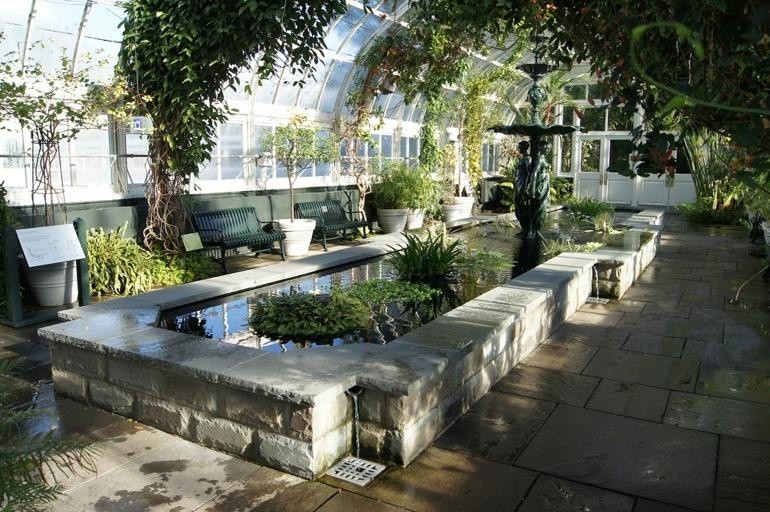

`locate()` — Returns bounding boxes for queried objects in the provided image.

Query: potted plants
[369,154,475,233]
[1,30,161,308]
[259,124,341,256]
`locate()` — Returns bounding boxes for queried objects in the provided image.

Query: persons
[512,139,532,238]
[532,138,555,239]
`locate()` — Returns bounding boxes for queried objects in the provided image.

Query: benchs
[191,207,286,274]
[294,200,369,251]
